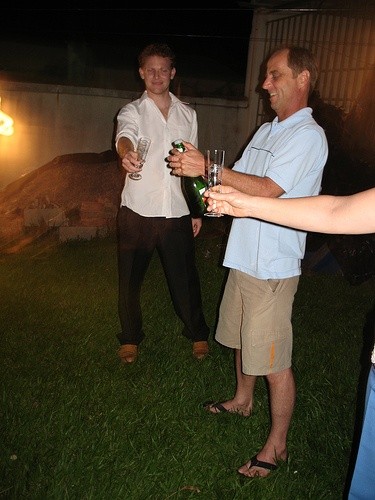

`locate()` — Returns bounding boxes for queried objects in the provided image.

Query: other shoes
[192,341,209,359]
[120,344,137,364]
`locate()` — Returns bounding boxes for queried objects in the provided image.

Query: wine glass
[128,137,152,180]
[204,149,226,216]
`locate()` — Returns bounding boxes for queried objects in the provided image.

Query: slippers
[235,453,290,479]
[202,399,233,415]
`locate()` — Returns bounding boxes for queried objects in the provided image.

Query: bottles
[173,140,209,219]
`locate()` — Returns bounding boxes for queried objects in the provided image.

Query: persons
[165,44,329,482]
[114,43,210,366]
[202,184,375,500]
